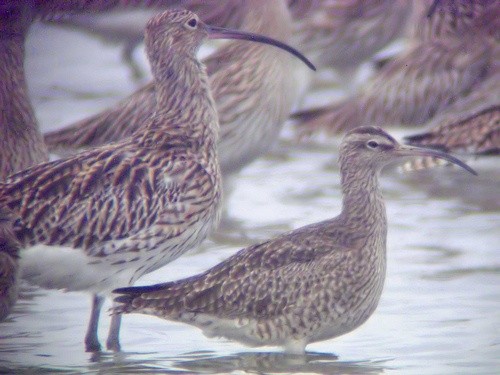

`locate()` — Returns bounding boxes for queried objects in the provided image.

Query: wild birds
[1,2,500,356]
